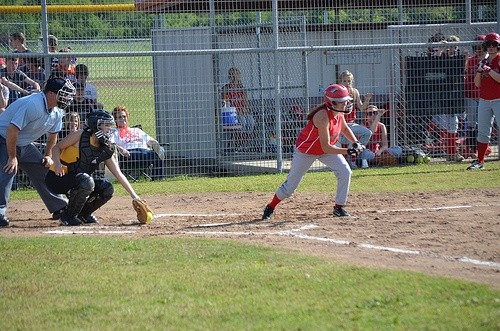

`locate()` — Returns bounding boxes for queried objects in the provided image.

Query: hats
[46,78,69,95]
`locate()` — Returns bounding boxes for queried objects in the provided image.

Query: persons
[358,105,402,159]
[338,69,374,169]
[426,33,492,161]
[44,109,154,226]
[223,68,256,153]
[110,107,166,180]
[0,74,76,226]
[466,33,500,170]
[0,30,97,145]
[262,84,366,220]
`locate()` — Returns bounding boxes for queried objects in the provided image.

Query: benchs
[224,125,243,148]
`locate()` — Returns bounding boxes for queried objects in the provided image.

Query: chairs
[121,124,170,183]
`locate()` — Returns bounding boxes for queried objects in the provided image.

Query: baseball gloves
[131,199,153,224]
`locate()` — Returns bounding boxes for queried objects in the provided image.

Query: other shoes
[0,219,9,225]
[53,205,67,218]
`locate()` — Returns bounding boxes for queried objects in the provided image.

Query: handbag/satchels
[378,147,422,165]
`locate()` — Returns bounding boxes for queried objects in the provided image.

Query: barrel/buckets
[221,107,238,125]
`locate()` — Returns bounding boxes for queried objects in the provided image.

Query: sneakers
[468,162,484,170]
[60,214,82,227]
[333,207,349,217]
[446,154,464,161]
[263,205,274,219]
[77,214,97,224]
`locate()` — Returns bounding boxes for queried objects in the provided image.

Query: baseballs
[407,155,414,162]
[415,157,423,164]
[146,211,152,222]
[424,157,430,162]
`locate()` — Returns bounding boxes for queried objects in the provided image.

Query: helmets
[89,110,114,147]
[472,35,487,51]
[324,85,354,108]
[483,32,500,46]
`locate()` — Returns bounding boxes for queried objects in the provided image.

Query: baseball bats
[352,142,362,150]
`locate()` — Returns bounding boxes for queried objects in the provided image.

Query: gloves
[348,141,365,156]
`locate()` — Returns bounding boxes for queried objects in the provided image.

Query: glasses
[367,111,378,116]
[485,41,499,47]
